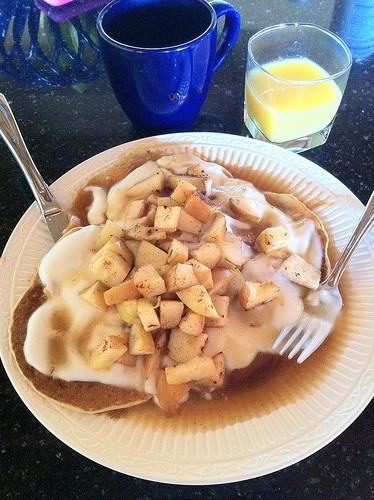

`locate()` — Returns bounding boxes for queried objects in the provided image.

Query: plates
[0,132,374,486]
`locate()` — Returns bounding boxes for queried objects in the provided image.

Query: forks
[271,191,374,365]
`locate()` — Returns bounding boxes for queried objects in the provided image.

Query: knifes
[0,93,70,244]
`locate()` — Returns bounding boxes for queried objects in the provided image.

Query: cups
[96,0,241,136]
[244,22,353,154]
